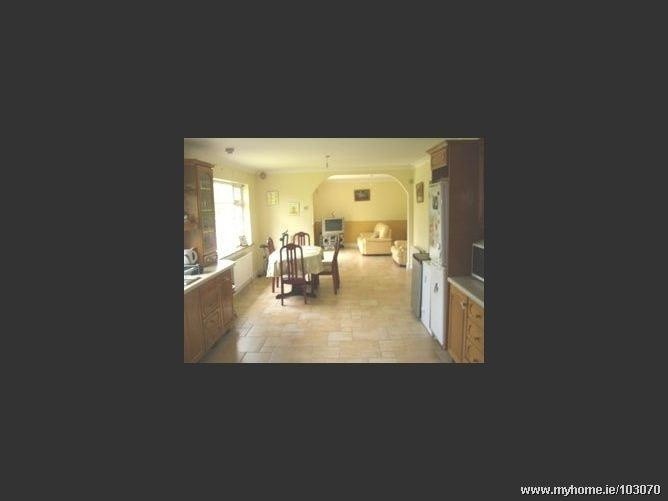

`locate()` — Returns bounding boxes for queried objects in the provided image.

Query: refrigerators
[428,180,449,342]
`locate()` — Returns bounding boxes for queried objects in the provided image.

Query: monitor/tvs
[323,216,344,233]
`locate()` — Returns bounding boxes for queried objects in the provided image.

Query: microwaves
[471,239,484,283]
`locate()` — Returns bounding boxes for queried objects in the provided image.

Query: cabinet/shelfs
[447,284,467,362]
[183,268,232,364]
[184,164,218,269]
[463,299,484,364]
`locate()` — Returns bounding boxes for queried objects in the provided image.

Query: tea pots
[184,248,198,266]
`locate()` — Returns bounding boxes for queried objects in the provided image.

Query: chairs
[266,232,343,308]
[358,223,393,256]
[394,239,407,268]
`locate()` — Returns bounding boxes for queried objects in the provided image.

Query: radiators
[231,252,253,293]
